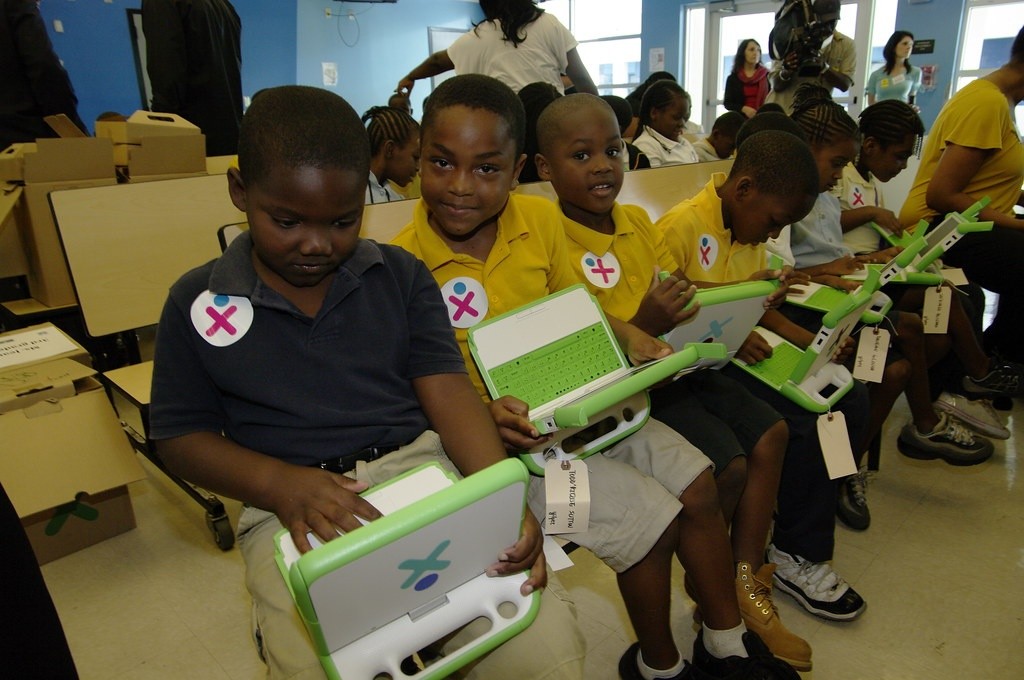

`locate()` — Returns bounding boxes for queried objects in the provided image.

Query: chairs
[1,153,740,666]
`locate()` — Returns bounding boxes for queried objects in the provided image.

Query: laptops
[272,192,994,680]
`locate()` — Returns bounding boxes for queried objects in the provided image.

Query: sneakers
[897,411,996,466]
[838,467,872,532]
[764,541,868,622]
[962,364,1024,397]
[936,392,1013,441]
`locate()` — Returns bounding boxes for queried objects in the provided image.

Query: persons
[142,0,244,157]
[764,0,857,117]
[145,85,585,680]
[357,27,1024,680]
[901,27,1024,359]
[867,31,922,115]
[396,0,598,98]
[723,39,772,118]
[0,0,93,154]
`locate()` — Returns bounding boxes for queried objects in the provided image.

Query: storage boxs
[0,321,148,566]
[0,110,240,309]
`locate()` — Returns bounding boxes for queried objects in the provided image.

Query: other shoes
[619,627,802,680]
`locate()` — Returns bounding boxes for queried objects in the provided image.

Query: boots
[692,554,813,668]
